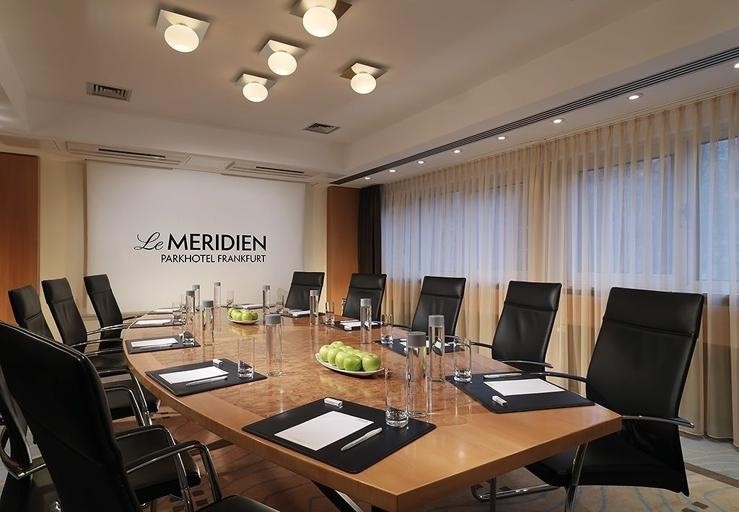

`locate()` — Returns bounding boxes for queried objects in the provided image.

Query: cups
[381,313,394,344]
[325,302,335,327]
[453,336,473,384]
[238,336,254,378]
[386,366,410,429]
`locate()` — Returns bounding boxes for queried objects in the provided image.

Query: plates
[315,352,385,380]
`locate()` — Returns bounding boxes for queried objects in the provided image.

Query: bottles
[265,314,283,375]
[407,333,432,417]
[174,282,283,348]
[429,315,446,382]
[359,297,374,343]
[309,290,319,326]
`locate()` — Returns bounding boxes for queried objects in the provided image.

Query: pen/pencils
[483,372,523,379]
[494,396,509,406]
[340,427,383,452]
[141,345,172,349]
[186,376,228,387]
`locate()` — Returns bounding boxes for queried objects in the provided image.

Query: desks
[116,299,625,510]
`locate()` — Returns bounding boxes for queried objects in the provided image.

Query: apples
[319,340,381,371]
[228,307,258,321]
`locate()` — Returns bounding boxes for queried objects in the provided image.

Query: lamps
[150,5,214,58]
[287,0,354,40]
[233,69,283,105]
[338,57,390,99]
[255,35,309,80]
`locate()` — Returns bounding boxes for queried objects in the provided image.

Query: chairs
[454,278,565,384]
[0,317,291,509]
[79,274,146,350]
[339,272,389,320]
[395,270,469,341]
[0,370,204,510]
[282,268,327,310]
[38,273,136,379]
[6,283,162,426]
[469,284,705,510]
